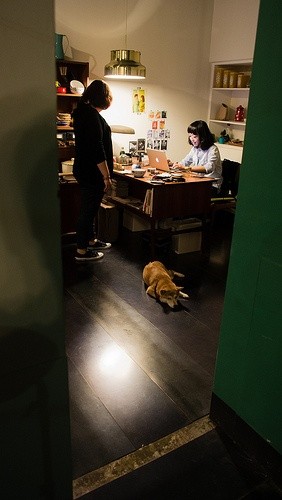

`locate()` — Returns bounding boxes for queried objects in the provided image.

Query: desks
[110,160,217,256]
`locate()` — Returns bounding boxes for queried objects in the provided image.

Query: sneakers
[75,249,104,261]
[88,239,111,249]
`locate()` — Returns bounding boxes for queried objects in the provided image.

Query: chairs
[211,160,241,209]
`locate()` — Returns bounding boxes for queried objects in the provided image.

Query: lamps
[110,125,135,134]
[103,0,146,79]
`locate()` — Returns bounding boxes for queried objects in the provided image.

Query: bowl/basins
[56,112,71,126]
[132,169,146,177]
[69,80,85,95]
[61,157,76,173]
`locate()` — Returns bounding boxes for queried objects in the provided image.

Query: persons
[72,80,114,260]
[171,120,223,194]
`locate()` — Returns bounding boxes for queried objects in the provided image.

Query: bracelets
[103,177,111,180]
[189,166,192,171]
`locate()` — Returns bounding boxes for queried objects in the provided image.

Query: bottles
[120,147,125,156]
[236,104,244,121]
[215,68,250,88]
[214,102,228,120]
[225,123,231,139]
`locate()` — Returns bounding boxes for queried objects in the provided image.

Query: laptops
[146,148,181,173]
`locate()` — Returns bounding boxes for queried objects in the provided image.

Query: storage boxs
[171,218,202,255]
[123,208,151,232]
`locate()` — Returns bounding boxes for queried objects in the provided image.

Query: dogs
[143,261,190,308]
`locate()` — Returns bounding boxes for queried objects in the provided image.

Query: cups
[218,137,224,144]
[56,87,66,94]
[113,155,140,171]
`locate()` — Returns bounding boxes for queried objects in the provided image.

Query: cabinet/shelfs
[207,0,260,164]
[56,60,89,234]
